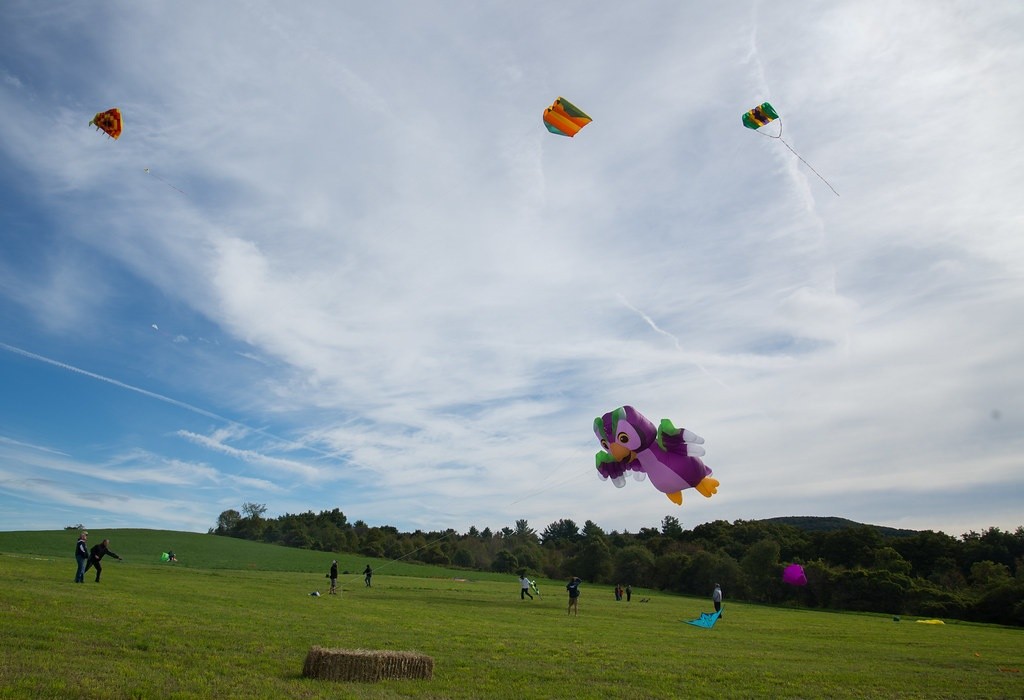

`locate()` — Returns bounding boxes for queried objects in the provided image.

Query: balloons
[593,404,721,506]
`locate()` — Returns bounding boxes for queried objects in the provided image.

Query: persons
[566,576,582,616]
[74,531,88,583]
[84,539,122,582]
[713,583,723,618]
[615,584,632,602]
[363,564,372,587]
[329,560,338,594]
[519,574,534,600]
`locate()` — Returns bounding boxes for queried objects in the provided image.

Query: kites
[742,101,779,130]
[677,603,726,629]
[89,107,122,140]
[529,580,540,596]
[784,564,808,585]
[542,96,593,137]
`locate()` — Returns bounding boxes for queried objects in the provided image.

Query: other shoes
[333,593,336,595]
[329,593,332,594]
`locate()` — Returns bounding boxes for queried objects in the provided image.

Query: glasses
[86,534,88,536]
